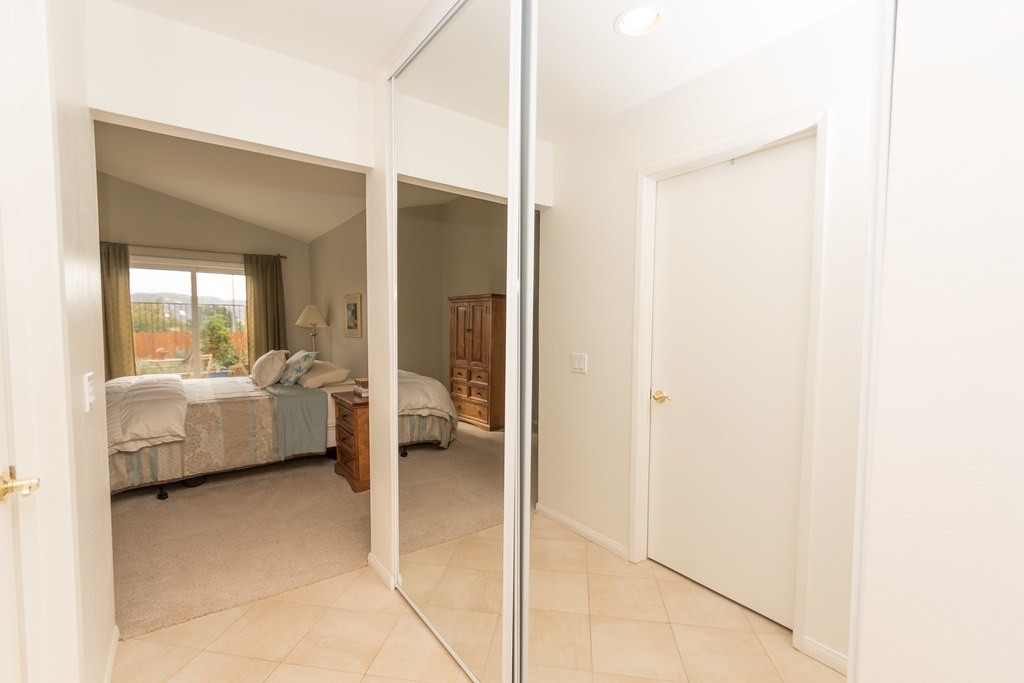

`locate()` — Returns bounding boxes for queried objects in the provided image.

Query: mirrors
[383,0,510,683]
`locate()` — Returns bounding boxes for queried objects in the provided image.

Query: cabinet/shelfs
[446,292,507,435]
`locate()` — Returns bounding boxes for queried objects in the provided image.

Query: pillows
[248,347,290,391]
[280,348,318,388]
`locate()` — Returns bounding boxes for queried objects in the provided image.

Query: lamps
[292,302,331,352]
[614,4,665,43]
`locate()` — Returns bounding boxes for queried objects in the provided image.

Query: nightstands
[330,387,371,496]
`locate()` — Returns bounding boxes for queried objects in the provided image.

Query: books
[353,386,369,398]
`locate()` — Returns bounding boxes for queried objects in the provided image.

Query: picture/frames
[340,291,364,340]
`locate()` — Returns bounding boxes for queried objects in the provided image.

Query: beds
[398,366,463,454]
[105,371,354,500]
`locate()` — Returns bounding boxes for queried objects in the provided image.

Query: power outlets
[82,370,101,409]
[568,354,588,377]
